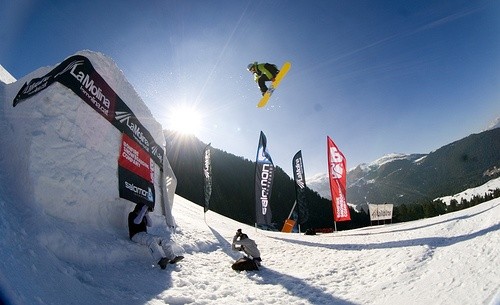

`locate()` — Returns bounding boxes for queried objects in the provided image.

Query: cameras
[236,229,242,236]
[146,203,154,212]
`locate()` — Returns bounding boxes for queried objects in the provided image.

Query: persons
[232,230,262,273]
[128,203,183,270]
[248,62,279,95]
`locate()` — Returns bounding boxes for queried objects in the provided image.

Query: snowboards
[257,62,293,107]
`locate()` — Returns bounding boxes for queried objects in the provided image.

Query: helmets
[247,63,254,71]
[240,234,247,240]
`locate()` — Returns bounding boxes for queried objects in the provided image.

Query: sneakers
[255,260,261,266]
[261,91,265,95]
[255,262,262,270]
[158,258,169,270]
[170,256,184,264]
